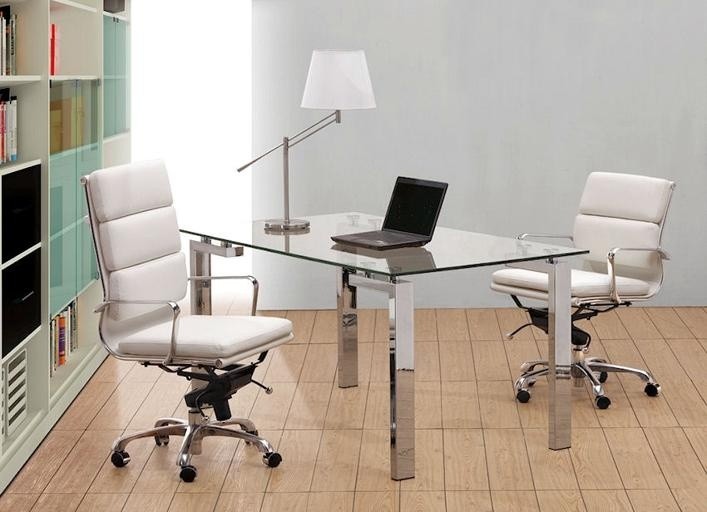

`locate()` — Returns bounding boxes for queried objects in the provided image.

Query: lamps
[237,50,377,233]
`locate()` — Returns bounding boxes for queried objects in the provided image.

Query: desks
[179,212,590,481]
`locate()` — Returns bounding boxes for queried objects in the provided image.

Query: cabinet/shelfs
[0,0,131,495]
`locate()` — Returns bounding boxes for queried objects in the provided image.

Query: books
[50,96,85,154]
[0,13,15,75]
[50,297,79,378]
[1,96,17,164]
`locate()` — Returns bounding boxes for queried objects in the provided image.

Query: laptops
[331,176,448,251]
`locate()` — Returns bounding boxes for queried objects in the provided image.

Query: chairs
[490,171,676,410]
[80,159,295,483]
